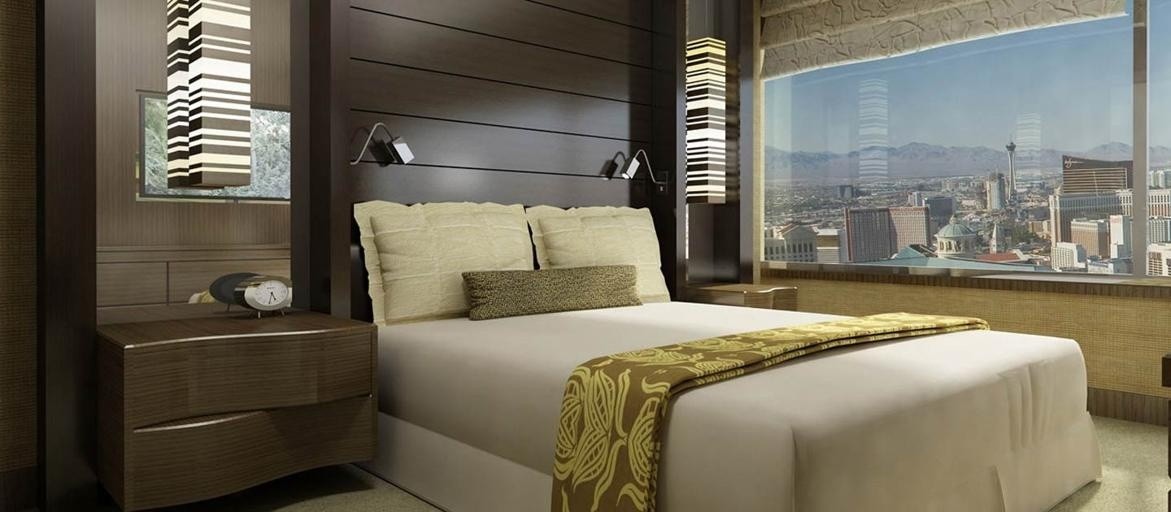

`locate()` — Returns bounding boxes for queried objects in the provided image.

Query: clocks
[235,274,295,320]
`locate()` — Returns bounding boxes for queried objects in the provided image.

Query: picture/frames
[140,96,290,200]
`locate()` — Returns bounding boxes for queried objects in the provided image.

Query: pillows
[525,205,674,306]
[461,265,643,320]
[352,199,533,329]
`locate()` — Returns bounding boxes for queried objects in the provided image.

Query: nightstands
[682,282,799,310]
[95,311,379,512]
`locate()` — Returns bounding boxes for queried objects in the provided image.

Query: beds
[366,304,1104,512]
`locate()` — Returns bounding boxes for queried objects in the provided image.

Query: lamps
[599,151,626,181]
[352,126,393,167]
[620,149,669,195]
[189,0,251,187]
[350,122,414,166]
[165,0,190,188]
[685,37,727,205]
